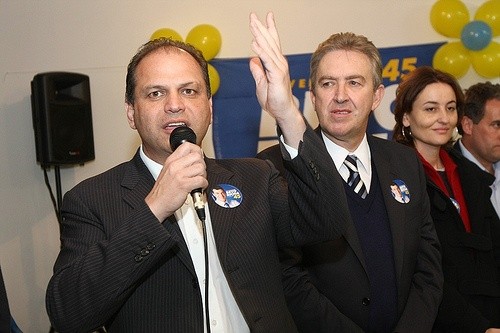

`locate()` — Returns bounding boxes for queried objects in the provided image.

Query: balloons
[150,28,184,44]
[429,0,500,80]
[185,24,222,62]
[207,64,220,95]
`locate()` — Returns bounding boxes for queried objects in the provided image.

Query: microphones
[170,127,205,221]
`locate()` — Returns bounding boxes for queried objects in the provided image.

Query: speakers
[30,71,95,170]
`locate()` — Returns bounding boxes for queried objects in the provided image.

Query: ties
[343,154,368,199]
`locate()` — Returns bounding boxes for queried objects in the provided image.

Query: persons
[210,185,240,207]
[254,32,443,333]
[390,65,500,333]
[46,9,348,333]
[391,182,410,204]
